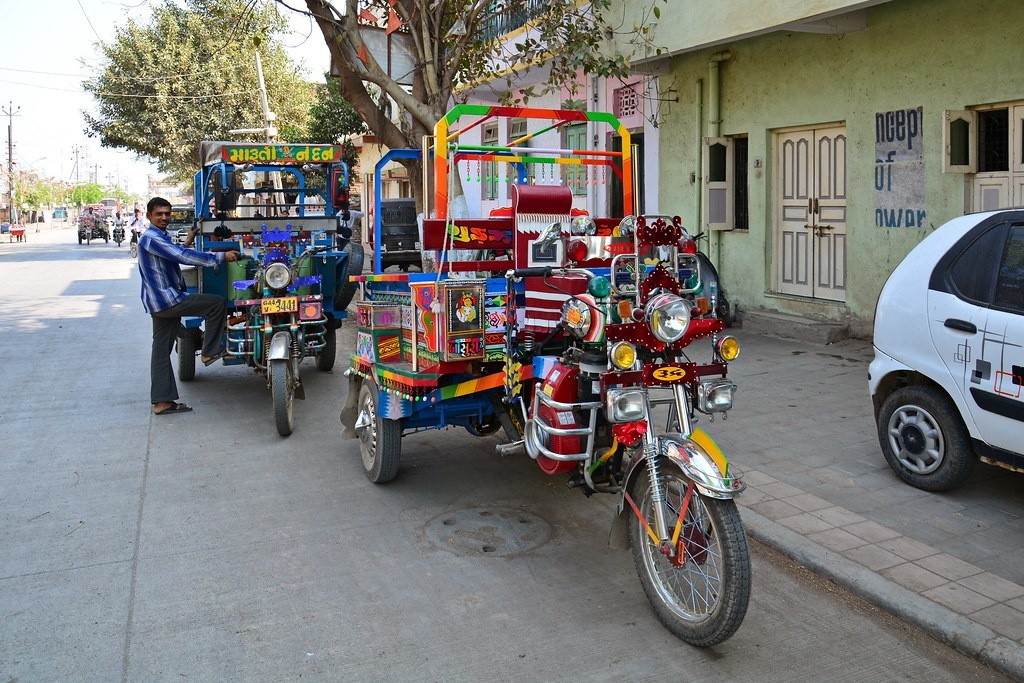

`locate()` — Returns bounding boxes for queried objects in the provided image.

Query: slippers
[156,400,192,414]
[205,349,228,366]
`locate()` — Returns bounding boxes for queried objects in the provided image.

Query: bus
[99,198,118,217]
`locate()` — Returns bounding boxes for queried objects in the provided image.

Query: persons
[112,212,127,241]
[335,205,371,251]
[130,207,148,247]
[85,207,96,220]
[137,197,241,416]
[282,207,300,217]
[16,214,25,242]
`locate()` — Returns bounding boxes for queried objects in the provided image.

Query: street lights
[71,144,84,181]
[0,101,23,197]
[106,172,115,184]
[90,163,102,184]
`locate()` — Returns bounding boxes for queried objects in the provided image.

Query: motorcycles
[110,220,128,248]
[76,209,109,245]
[339,101,751,649]
[175,140,365,439]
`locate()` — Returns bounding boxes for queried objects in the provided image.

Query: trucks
[166,203,194,242]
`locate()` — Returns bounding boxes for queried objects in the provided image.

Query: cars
[868,207,1024,491]
